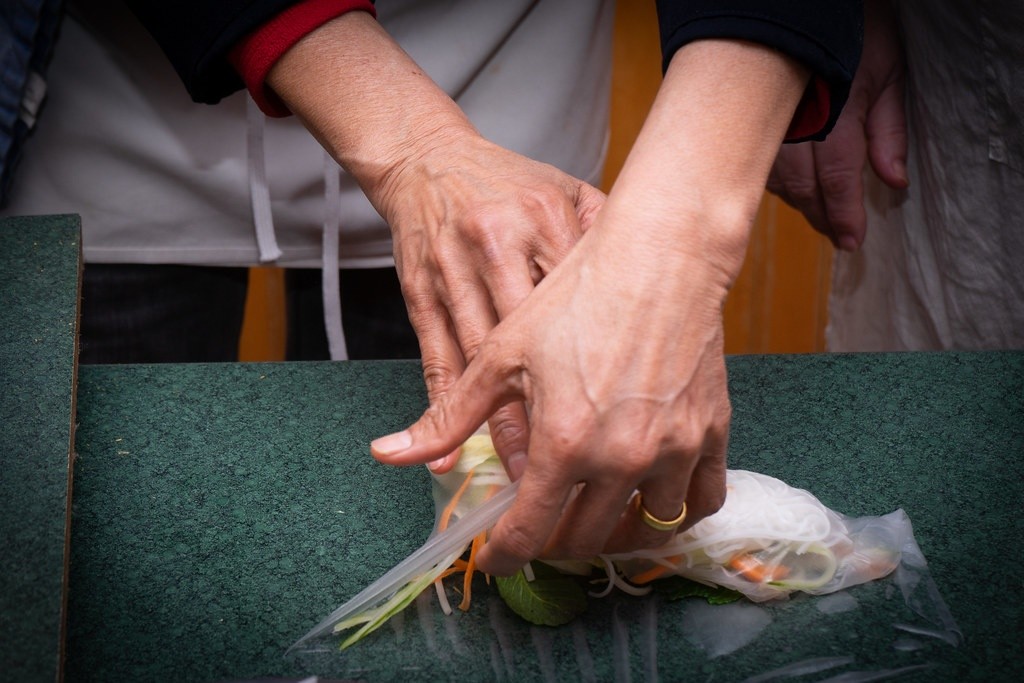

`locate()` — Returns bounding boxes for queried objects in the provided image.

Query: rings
[631,489,687,530]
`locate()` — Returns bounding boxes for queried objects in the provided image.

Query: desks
[0,212,1024,683]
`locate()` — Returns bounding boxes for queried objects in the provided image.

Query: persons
[766,0,1024,354]
[10,2,869,583]
